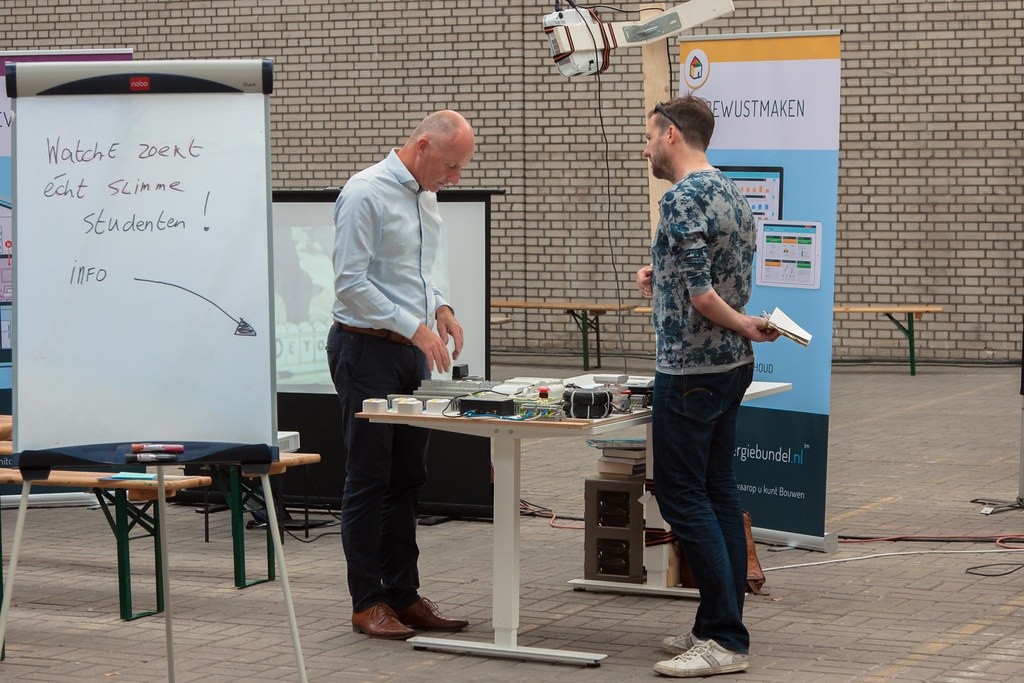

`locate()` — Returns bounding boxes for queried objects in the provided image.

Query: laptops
[243,485,336,530]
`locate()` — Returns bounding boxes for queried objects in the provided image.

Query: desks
[356,374,792,669]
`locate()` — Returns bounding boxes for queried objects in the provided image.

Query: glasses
[653,101,683,132]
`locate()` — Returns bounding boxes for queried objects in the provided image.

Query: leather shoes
[351,600,416,639]
[398,595,468,631]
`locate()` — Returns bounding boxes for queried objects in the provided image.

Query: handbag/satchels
[678,509,770,596]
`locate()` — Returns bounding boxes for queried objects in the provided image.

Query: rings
[644,288,647,295]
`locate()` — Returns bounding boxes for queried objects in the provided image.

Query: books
[761,306,813,347]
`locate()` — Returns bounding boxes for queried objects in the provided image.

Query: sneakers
[653,638,750,677]
[661,629,700,653]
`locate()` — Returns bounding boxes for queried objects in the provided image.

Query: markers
[128,442,184,452]
[125,453,179,462]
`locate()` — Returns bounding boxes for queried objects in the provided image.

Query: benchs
[490,300,641,371]
[0,414,321,621]
[635,306,944,376]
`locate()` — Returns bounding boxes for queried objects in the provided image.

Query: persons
[325,110,475,639]
[635,95,780,677]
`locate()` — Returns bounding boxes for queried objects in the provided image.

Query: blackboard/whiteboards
[10,89,282,470]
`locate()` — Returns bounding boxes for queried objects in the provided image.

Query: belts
[334,320,415,347]
